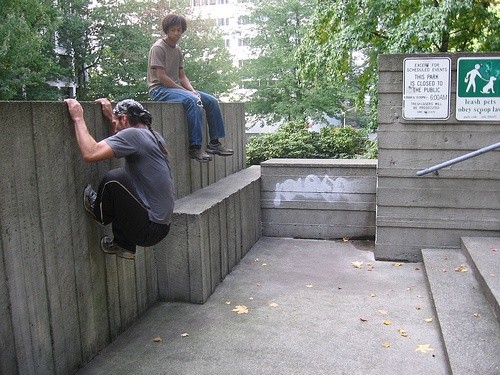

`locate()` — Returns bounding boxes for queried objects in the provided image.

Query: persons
[66,97,173,259]
[146,14,234,162]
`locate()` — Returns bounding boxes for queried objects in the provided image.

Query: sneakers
[206,142,234,156]
[189,146,212,161]
[83,185,104,226]
[101,235,137,260]
